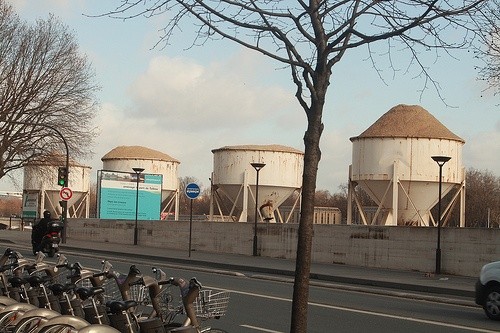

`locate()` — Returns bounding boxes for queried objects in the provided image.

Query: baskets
[0,258,72,289]
[125,273,151,303]
[151,286,182,326]
[184,289,229,318]
[81,271,123,305]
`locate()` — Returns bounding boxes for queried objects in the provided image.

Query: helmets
[43,211,51,218]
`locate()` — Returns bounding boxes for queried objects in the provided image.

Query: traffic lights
[59,201,65,208]
[57,166,67,186]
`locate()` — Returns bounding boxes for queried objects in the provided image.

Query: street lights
[431,155,452,275]
[132,167,145,246]
[249,161,266,259]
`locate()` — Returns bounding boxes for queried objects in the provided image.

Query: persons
[32,211,54,252]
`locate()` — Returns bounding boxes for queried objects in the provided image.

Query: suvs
[473,262,500,321]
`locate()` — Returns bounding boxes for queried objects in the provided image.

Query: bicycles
[0,247,230,333]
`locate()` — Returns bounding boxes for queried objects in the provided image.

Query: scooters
[31,220,63,259]
